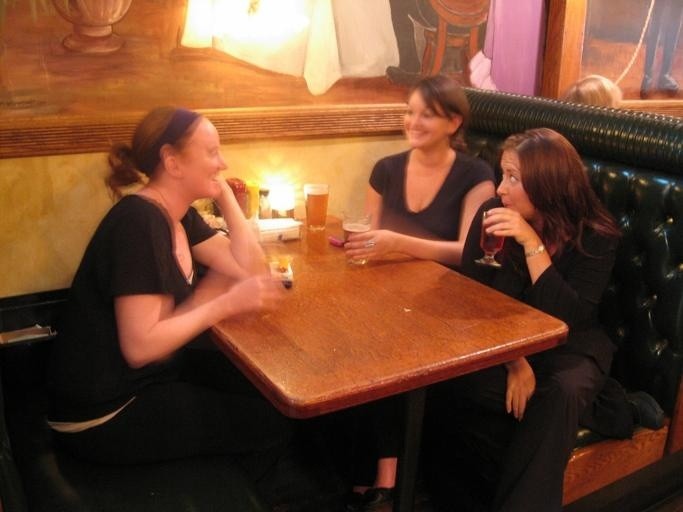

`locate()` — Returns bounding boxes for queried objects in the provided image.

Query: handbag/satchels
[580,378,664,437]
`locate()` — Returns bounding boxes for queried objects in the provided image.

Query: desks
[189,215,570,512]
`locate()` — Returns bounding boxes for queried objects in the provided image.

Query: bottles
[229,178,272,219]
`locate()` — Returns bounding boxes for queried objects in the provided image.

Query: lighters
[275,255,291,272]
[328,235,344,248]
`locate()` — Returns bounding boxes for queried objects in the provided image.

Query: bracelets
[523,243,545,258]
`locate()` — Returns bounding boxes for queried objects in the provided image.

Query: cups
[303,183,330,231]
[340,221,371,267]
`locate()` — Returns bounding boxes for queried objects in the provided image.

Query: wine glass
[475,211,507,268]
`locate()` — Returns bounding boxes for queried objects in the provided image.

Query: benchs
[455,85,683,512]
[0,324,271,512]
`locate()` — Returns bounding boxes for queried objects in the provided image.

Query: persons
[561,73,625,111]
[345,69,496,511]
[42,103,346,510]
[379,0,486,90]
[637,0,682,101]
[418,128,623,510]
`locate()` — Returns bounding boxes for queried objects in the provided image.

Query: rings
[363,238,375,248]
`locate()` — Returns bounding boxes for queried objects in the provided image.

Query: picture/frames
[540,0,683,117]
